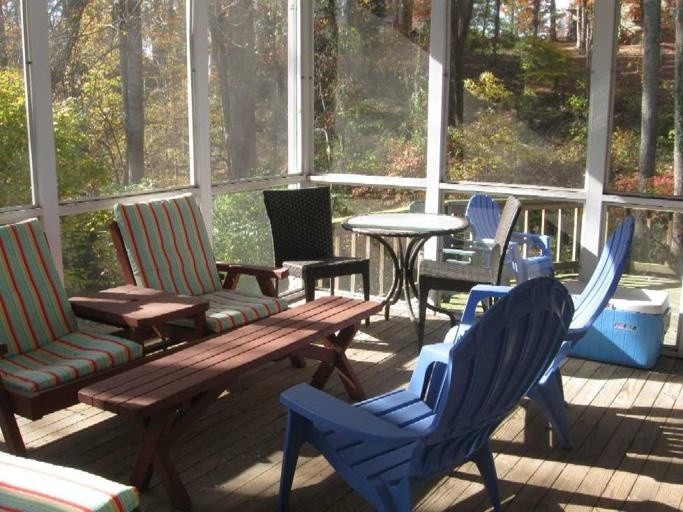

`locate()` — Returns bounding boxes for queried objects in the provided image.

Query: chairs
[104,192,307,413]
[406,214,637,450]
[416,196,523,355]
[262,186,372,329]
[276,275,574,512]
[465,193,556,289]
[2,450,141,511]
[0,217,146,458]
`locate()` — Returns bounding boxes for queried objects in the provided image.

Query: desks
[340,213,470,327]
[67,285,211,427]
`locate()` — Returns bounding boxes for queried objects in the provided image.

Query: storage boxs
[561,279,672,370]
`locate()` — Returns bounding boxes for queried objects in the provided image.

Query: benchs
[78,293,383,510]
